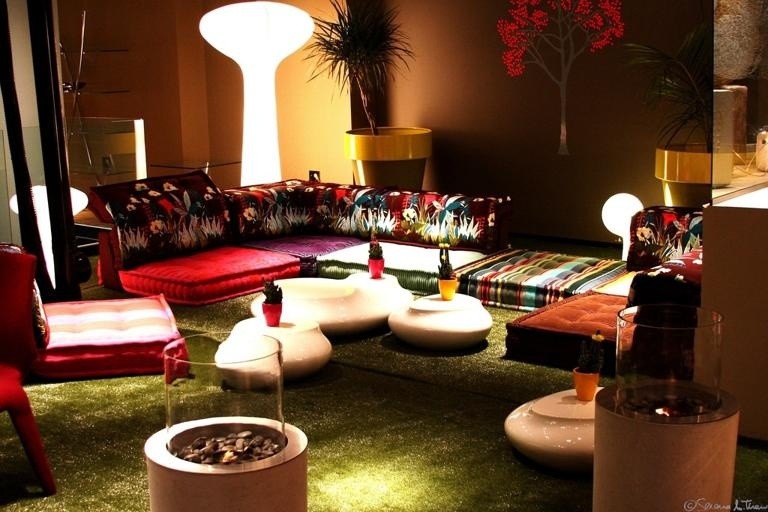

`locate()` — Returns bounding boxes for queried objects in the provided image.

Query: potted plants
[303,0,433,191]
[620,12,713,210]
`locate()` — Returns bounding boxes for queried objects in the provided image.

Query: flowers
[576,328,604,371]
[436,242,457,280]
[259,276,285,303]
[368,236,385,259]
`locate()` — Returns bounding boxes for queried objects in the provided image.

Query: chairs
[0,245,60,497]
[5,245,189,407]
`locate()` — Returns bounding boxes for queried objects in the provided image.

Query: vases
[262,303,283,329]
[438,281,460,302]
[367,257,385,280]
[575,368,598,402]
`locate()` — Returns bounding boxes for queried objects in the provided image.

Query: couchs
[452,204,705,375]
[86,169,304,311]
[318,189,511,295]
[228,177,381,275]
